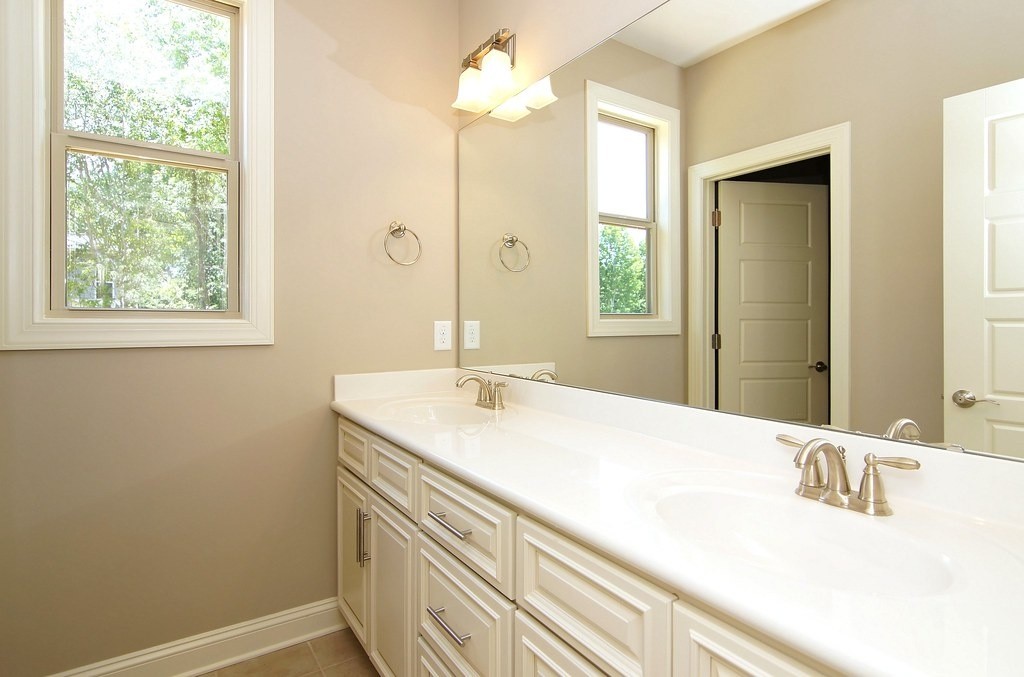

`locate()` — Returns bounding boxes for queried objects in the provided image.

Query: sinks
[370,394,513,436]
[644,484,884,580]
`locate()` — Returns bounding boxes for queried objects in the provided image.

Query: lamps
[488,72,559,124]
[451,27,518,114]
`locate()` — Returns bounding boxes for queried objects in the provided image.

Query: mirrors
[455,0,1024,462]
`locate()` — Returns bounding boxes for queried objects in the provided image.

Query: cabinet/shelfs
[336,415,422,677]
[515,515,679,677]
[672,599,827,676]
[417,461,517,677]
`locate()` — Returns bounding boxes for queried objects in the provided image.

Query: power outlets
[433,320,451,351]
[464,321,480,350]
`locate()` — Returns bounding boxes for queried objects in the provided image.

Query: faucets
[792,438,853,501]
[883,415,920,441]
[453,372,492,406]
[529,367,557,383]
